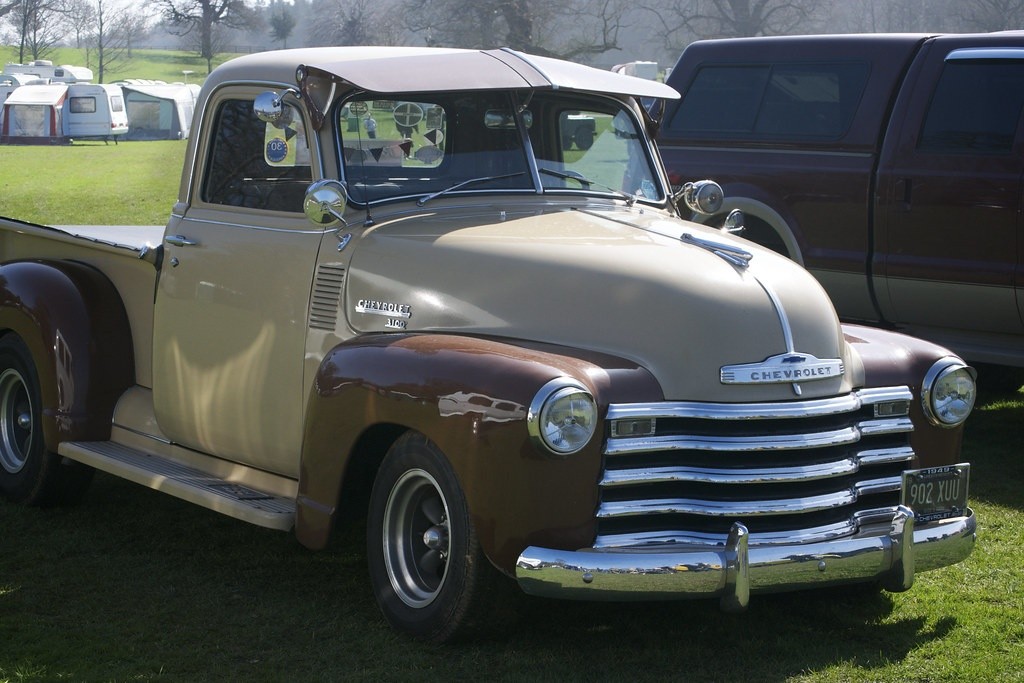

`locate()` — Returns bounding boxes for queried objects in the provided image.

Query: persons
[610,64,660,202]
[364,113,377,139]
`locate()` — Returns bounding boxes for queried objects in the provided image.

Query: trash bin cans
[346,117,359,133]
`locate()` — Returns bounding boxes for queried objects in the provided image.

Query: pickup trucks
[0,44,981,644]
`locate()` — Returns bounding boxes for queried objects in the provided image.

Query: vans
[622,31,1024,373]
